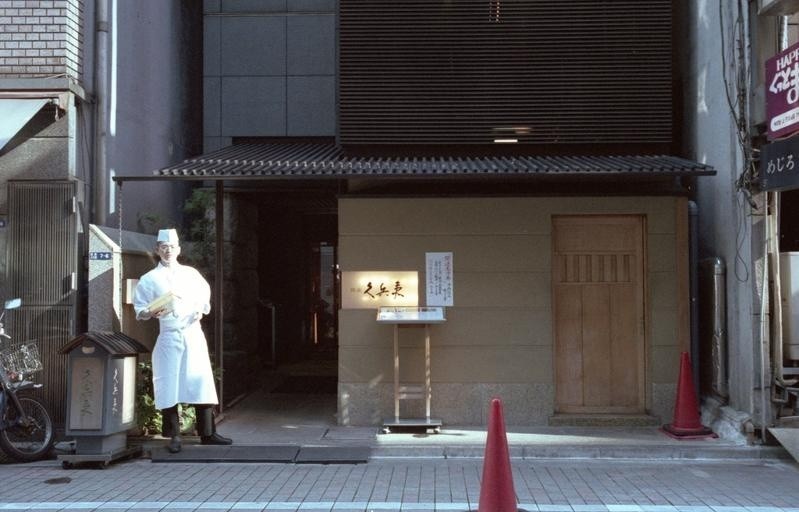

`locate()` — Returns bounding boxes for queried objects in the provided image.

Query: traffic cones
[664,350,713,435]
[478,398,519,512]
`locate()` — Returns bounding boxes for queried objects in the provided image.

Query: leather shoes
[169,439,181,452]
[201,432,232,445]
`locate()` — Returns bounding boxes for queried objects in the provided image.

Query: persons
[132,227,233,453]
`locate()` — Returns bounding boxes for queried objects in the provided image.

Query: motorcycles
[0,297,55,461]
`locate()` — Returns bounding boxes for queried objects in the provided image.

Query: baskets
[0,339,43,381]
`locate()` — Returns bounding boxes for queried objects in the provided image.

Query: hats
[157,229,179,242]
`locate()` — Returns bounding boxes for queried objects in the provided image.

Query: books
[144,291,183,314]
